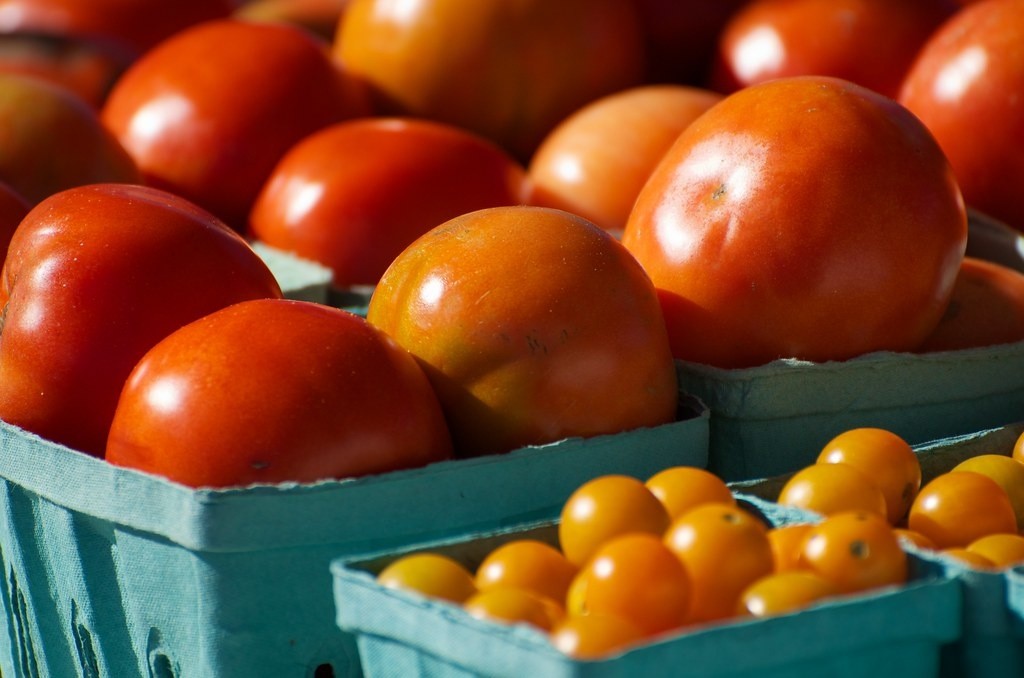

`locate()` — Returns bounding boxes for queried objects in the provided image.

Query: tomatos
[773,427,1024,576]
[368,463,906,661]
[0,0,1024,491]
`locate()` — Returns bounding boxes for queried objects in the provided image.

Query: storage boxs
[0,210,1024,678]
